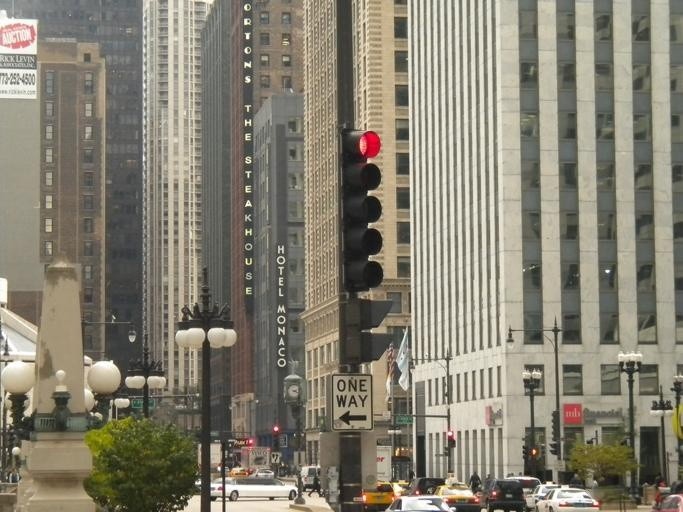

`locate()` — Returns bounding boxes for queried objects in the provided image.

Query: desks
[172,263,239,511]
[648,383,674,487]
[0,312,168,511]
[501,313,565,482]
[519,367,540,477]
[616,349,643,499]
[404,348,459,477]
[383,423,403,487]
[670,372,682,452]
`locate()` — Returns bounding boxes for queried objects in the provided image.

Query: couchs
[521,442,529,459]
[443,447,448,456]
[531,445,537,457]
[271,424,280,433]
[547,441,560,461]
[334,128,386,299]
[549,410,561,442]
[447,430,454,441]
[245,437,255,445]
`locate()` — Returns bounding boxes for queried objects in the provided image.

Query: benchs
[396,329,409,390]
[386,344,395,401]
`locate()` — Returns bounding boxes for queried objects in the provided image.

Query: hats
[362,475,602,512]
[652,489,682,512]
[193,463,321,501]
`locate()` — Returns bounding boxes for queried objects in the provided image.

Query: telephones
[330,375,374,434]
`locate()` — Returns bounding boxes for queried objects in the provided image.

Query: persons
[468,471,481,490]
[308,476,322,497]
[407,467,415,486]
[482,473,491,488]
